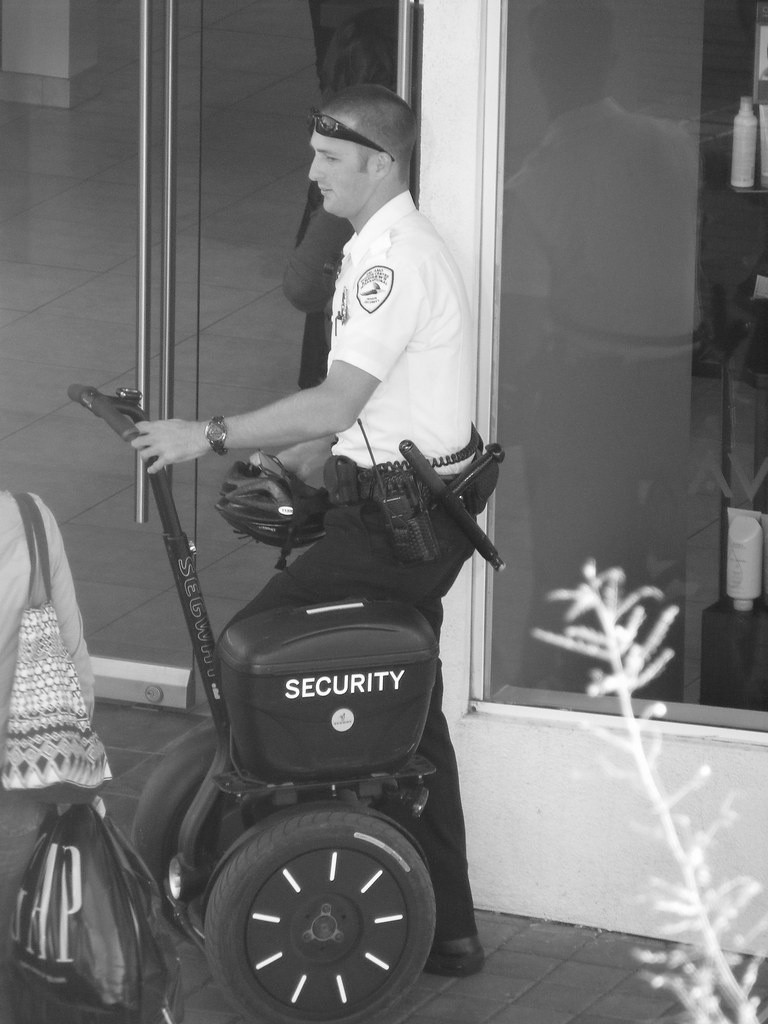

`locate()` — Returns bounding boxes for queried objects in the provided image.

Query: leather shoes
[421,933,485,976]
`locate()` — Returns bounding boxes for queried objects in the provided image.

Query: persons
[288,1,719,699]
[0,491,121,1007]
[132,84,485,980]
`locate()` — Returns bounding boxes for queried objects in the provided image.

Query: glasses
[304,107,396,162]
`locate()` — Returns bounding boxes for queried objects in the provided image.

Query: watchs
[204,416,228,454]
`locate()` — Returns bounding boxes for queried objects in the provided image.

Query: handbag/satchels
[12,802,167,1024]
[1,492,113,807]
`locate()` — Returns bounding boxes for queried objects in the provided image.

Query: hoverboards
[67,380,443,1024]
